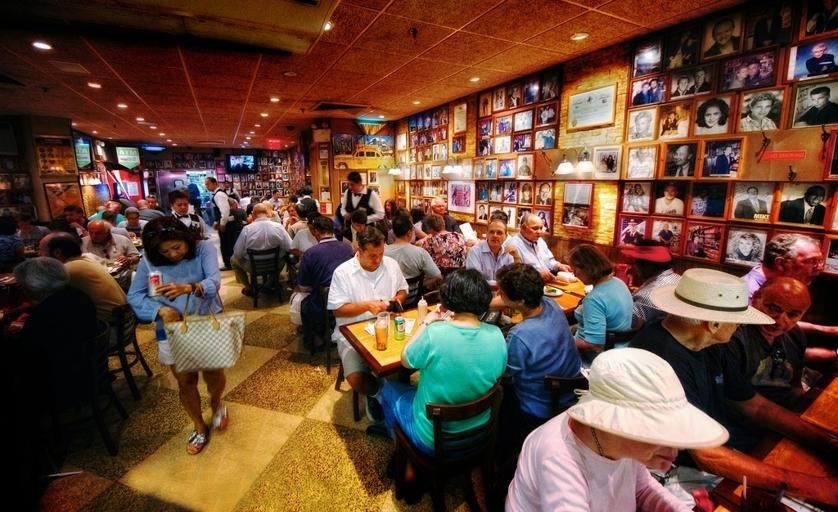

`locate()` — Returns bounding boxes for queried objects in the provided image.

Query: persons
[704,16,739,57]
[685,68,711,93]
[745,63,771,89]
[1,194,164,465]
[205,177,241,271]
[694,99,729,135]
[395,69,589,234]
[632,111,652,140]
[724,63,749,89]
[128,217,229,455]
[794,86,837,125]
[671,144,693,177]
[741,92,778,130]
[661,105,690,138]
[232,170,681,482]
[632,83,652,103]
[669,33,697,68]
[804,43,838,75]
[508,233,836,512]
[645,79,663,101]
[167,190,218,240]
[714,145,734,176]
[217,152,301,200]
[671,74,689,96]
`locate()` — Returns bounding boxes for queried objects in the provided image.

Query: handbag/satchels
[164,311,245,372]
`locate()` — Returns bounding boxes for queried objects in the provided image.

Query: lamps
[388,162,403,176]
[442,155,464,174]
[555,152,592,175]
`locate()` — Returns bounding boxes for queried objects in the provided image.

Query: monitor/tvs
[225,153,258,173]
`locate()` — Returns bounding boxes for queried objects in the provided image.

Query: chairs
[89,302,152,456]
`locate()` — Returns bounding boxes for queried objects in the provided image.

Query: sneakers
[385,450,408,478]
[242,288,261,298]
[220,267,232,271]
[365,425,388,436]
[365,396,384,422]
[303,337,321,349]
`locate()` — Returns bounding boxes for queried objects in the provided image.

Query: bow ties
[354,193,361,196]
[177,216,188,218]
[211,193,216,196]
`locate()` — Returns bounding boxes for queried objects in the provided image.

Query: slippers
[186,423,209,455]
[211,403,228,431]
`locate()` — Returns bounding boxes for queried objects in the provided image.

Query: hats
[650,268,777,326]
[618,246,672,262]
[567,348,730,450]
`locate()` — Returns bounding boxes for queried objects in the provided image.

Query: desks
[712,374,838,512]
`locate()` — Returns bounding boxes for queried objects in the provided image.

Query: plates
[542,285,562,297]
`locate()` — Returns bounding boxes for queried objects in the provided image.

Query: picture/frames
[591,146,623,180]
[566,83,618,133]
[561,183,595,229]
[472,67,562,236]
[452,102,468,153]
[408,103,448,215]
[320,150,328,159]
[397,180,406,209]
[447,180,475,215]
[340,170,381,204]
[144,150,311,203]
[396,132,407,162]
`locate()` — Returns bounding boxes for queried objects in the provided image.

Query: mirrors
[317,142,333,216]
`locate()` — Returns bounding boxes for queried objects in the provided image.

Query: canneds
[395,316,405,342]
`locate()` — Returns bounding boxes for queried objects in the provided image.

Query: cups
[373,312,391,351]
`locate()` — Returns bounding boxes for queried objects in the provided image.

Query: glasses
[142,228,175,239]
[103,247,111,259]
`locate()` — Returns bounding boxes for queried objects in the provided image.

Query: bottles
[416,296,427,318]
[394,303,405,342]
